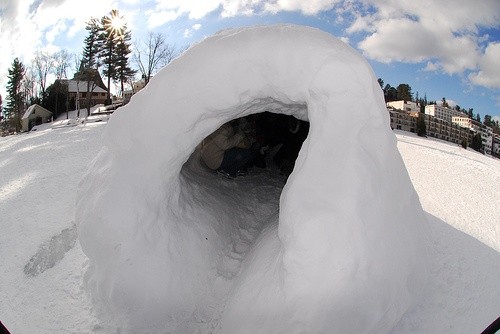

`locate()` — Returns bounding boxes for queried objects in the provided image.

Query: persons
[201,111,309,181]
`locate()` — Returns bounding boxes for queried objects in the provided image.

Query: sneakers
[217,170,233,180]
[236,170,248,176]
